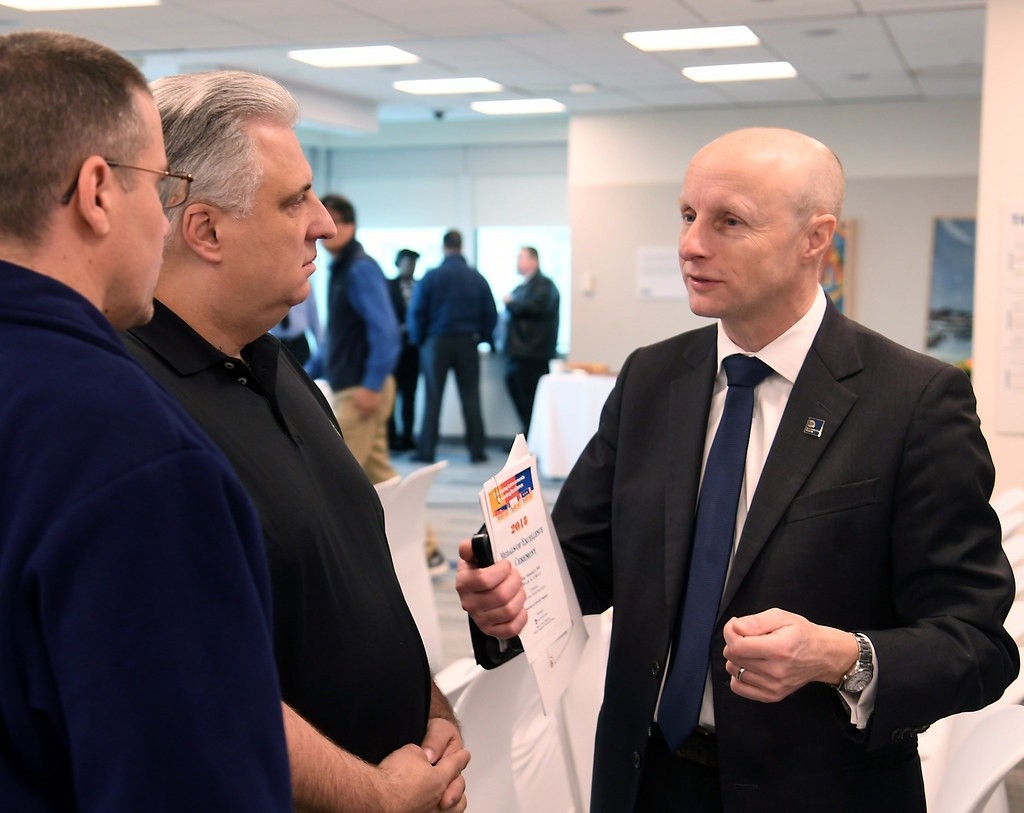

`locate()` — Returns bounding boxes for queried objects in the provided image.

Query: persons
[0,29,293,813]
[320,194,450,577]
[110,70,470,813]
[456,127,1020,813]
[267,283,322,367]
[503,248,559,441]
[385,249,419,451]
[406,232,497,463]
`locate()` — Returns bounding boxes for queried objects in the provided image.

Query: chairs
[917,647,1024,760]
[453,652,585,813]
[929,706,1024,813]
[1002,488,1024,643]
[373,458,455,672]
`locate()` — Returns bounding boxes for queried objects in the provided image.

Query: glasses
[61,160,197,208]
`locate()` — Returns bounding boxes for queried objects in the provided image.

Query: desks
[527,370,617,481]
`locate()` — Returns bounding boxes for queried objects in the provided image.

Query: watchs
[837,633,875,695]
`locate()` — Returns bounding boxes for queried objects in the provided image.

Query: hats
[395,249,420,266]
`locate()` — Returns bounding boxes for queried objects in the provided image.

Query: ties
[657,354,775,742]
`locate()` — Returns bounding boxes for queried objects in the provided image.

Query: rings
[736,668,745,680]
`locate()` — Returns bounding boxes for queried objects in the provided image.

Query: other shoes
[401,438,414,449]
[409,455,432,463]
[472,454,486,463]
